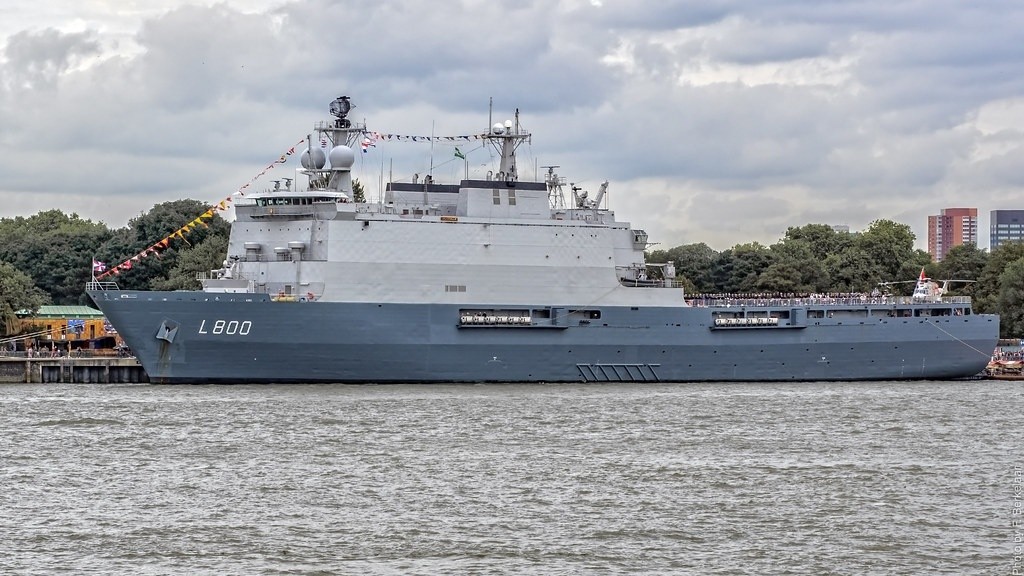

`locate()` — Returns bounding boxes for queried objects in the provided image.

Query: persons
[0,344,82,358]
[684,292,881,307]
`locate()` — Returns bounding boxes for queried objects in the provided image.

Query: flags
[362,143,368,154]
[454,147,464,160]
[365,137,376,148]
[94,258,107,272]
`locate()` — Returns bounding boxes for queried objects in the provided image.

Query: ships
[85,95,1001,388]
